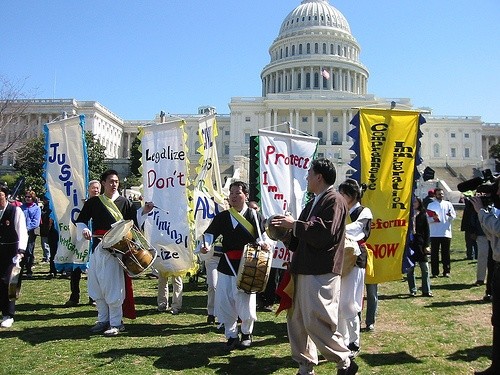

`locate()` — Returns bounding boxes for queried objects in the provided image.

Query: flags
[321,67,330,80]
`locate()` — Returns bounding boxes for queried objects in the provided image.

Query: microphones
[457,177,483,192]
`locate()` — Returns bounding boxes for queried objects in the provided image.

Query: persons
[272,159,359,375]
[75,170,154,337]
[192,181,274,351]
[0,158,456,375]
[461,175,500,375]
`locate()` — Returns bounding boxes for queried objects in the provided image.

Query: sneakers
[91,321,125,335]
[170,306,180,315]
[157,304,169,311]
[0,315,15,328]
[206,315,217,324]
[336,356,358,375]
[224,333,252,350]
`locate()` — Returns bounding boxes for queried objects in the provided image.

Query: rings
[279,221,282,225]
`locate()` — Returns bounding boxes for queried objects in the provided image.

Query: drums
[234,242,275,295]
[8,266,22,301]
[265,214,289,242]
[100,219,159,277]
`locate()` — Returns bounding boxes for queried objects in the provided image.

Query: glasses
[25,194,32,199]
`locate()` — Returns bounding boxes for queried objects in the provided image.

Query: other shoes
[422,293,434,297]
[348,342,362,358]
[483,293,493,302]
[366,324,374,331]
[63,299,79,307]
[429,275,440,278]
[474,280,485,287]
[443,273,452,278]
[464,257,478,260]
[409,292,415,295]
